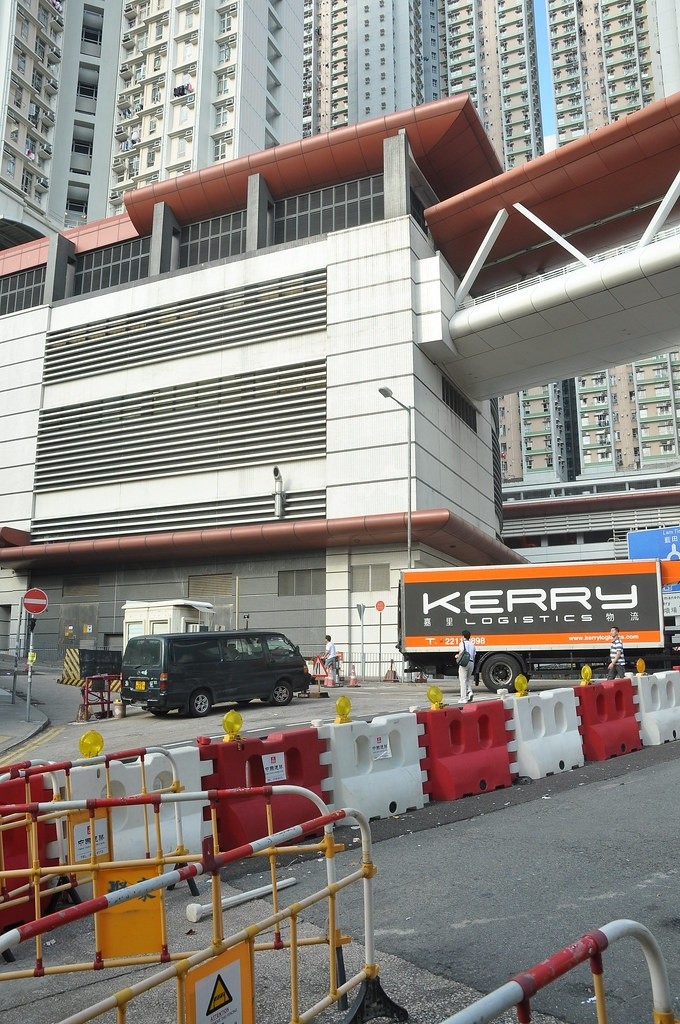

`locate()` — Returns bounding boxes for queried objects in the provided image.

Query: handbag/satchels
[456,642,470,667]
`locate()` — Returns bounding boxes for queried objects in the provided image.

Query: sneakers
[458,699,467,704]
[468,693,475,701]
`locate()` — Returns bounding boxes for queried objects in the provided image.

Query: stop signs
[23,586,49,615]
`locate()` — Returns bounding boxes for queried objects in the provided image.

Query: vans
[119,628,312,719]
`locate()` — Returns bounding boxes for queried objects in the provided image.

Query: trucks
[394,557,680,694]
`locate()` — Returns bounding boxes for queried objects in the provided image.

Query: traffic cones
[326,663,336,688]
[320,665,328,687]
[348,665,361,687]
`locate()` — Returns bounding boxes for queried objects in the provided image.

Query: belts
[469,660,473,662]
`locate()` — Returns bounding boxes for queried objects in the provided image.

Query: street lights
[377,386,412,569]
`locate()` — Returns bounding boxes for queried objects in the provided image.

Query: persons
[607,627,625,680]
[455,630,476,705]
[323,635,336,668]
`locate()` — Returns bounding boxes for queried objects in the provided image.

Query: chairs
[226,644,242,661]
